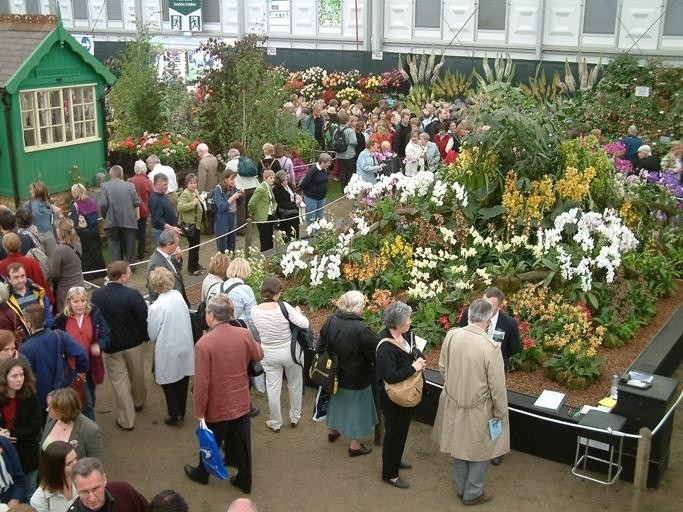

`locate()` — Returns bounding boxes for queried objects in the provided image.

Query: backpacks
[205,184,223,216]
[333,127,347,152]
[260,159,275,180]
[236,157,259,177]
[290,318,314,362]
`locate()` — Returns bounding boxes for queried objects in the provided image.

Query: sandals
[190,265,206,276]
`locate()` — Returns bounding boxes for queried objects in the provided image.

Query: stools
[570,409,630,510]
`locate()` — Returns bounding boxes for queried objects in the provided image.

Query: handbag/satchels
[89,352,105,384]
[309,347,339,394]
[76,214,88,232]
[277,205,299,220]
[312,386,329,422]
[195,422,228,480]
[178,221,196,238]
[68,375,88,412]
[25,248,52,281]
[384,371,424,408]
[189,302,209,342]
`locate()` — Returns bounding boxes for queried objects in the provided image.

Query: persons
[636,145,659,176]
[431,297,511,506]
[0,143,427,512]
[283,92,461,195]
[660,140,683,180]
[621,125,644,162]
[460,288,521,465]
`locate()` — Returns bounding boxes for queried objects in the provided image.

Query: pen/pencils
[564,406,581,418]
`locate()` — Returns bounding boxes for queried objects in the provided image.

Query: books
[531,371,655,416]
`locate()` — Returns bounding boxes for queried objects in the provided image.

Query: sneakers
[250,407,261,417]
[331,176,339,182]
[266,420,281,432]
[463,490,492,505]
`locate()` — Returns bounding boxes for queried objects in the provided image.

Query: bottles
[610,374,620,399]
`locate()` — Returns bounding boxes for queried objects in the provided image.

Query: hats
[327,107,337,114]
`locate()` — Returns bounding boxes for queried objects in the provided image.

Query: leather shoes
[328,432,340,442]
[400,462,411,470]
[349,442,369,457]
[165,414,182,424]
[389,477,409,488]
[184,464,208,484]
